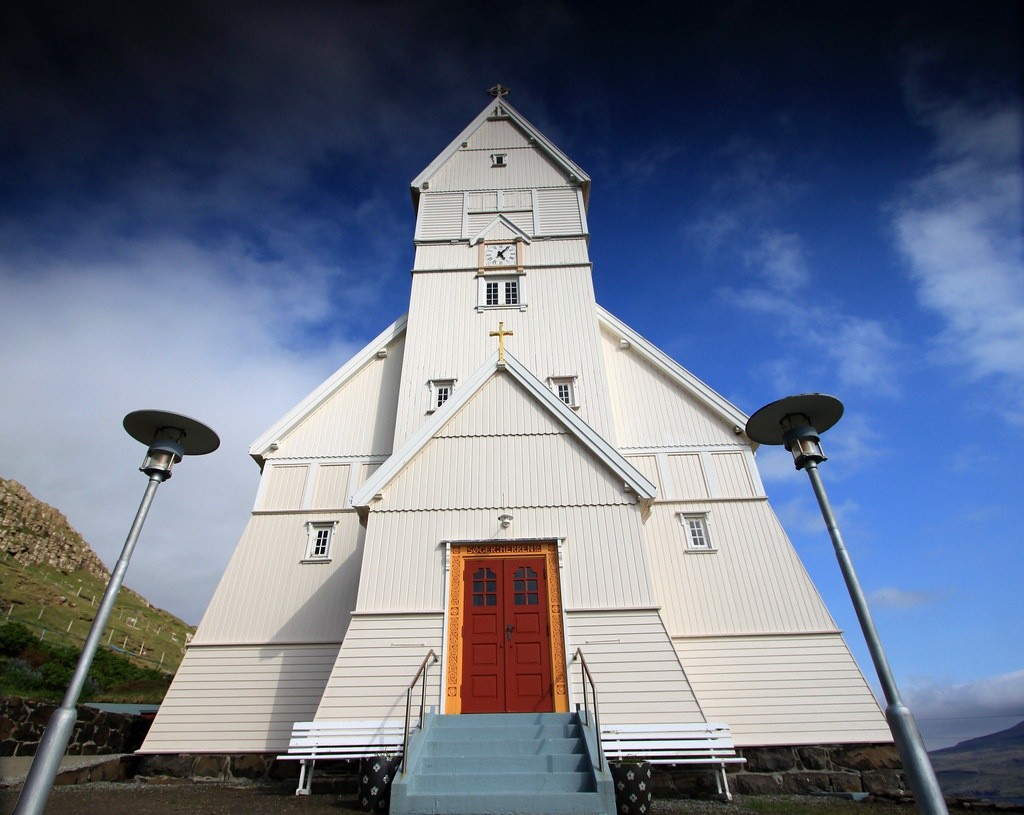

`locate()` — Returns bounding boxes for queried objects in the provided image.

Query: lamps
[498,514,513,527]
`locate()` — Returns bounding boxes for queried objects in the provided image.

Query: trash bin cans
[359,755,401,814]
[608,761,652,815]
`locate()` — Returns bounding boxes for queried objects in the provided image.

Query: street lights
[12,410,220,815]
[745,393,949,815]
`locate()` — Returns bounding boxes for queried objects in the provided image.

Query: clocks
[478,237,523,273]
